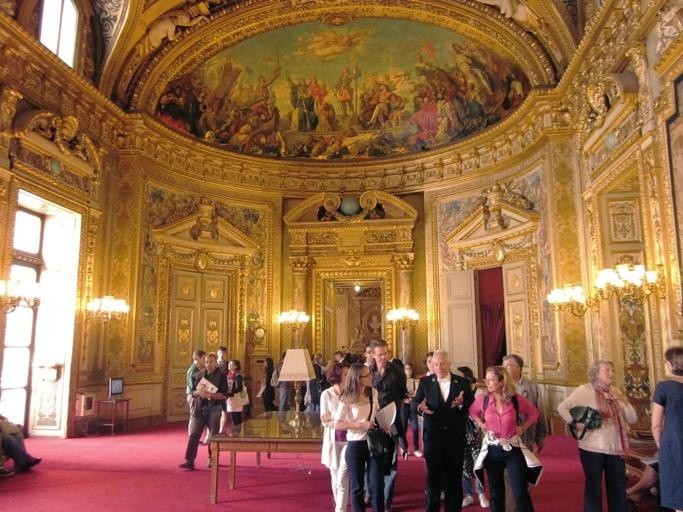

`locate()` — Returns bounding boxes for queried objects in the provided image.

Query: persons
[649,345,683,512]
[0,414,42,472]
[556,358,638,512]
[626,452,660,509]
[320,337,549,512]
[179,347,329,470]
[0,447,15,477]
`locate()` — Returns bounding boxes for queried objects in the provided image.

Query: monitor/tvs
[109,376,124,400]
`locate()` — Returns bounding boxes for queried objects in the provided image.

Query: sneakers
[462,496,473,507]
[0,457,42,478]
[478,492,489,508]
[180,463,194,468]
[414,451,423,457]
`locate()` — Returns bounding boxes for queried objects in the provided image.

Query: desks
[210,411,325,504]
[95,398,132,436]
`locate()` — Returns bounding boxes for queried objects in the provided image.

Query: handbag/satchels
[234,387,250,405]
[569,406,602,430]
[367,428,389,458]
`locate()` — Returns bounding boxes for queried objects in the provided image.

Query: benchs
[626,448,655,489]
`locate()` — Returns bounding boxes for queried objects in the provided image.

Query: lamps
[85,296,129,325]
[387,308,419,329]
[276,310,310,331]
[0,279,44,314]
[546,285,600,317]
[354,284,360,292]
[591,263,665,306]
[278,348,317,425]
